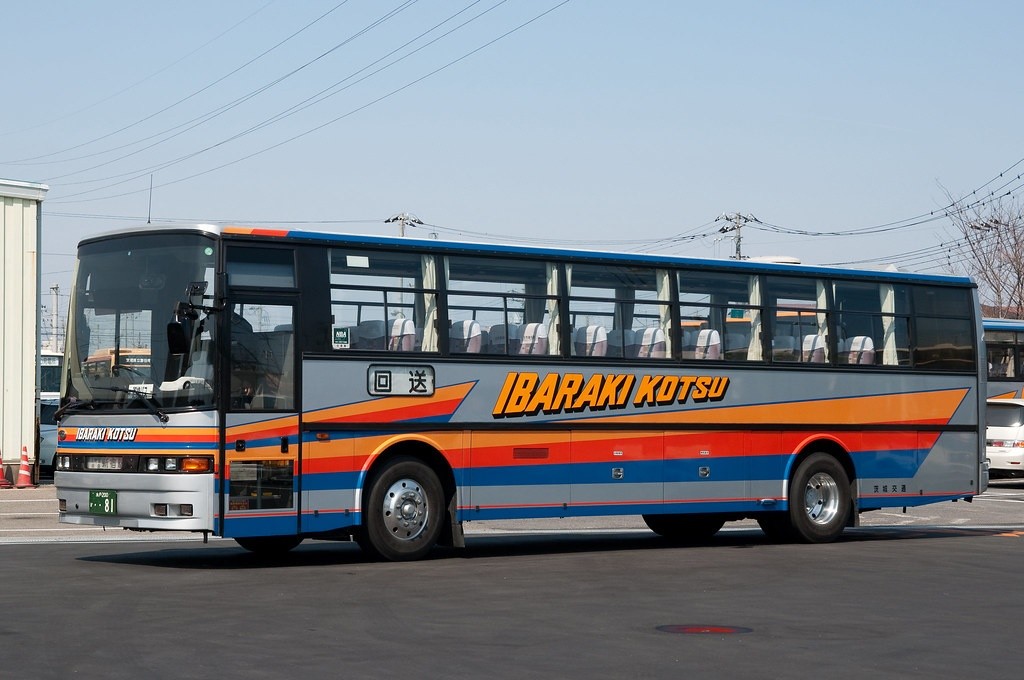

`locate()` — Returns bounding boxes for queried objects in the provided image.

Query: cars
[38,399,60,480]
[985,398,1024,481]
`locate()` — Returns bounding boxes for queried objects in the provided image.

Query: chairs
[254,316,876,410]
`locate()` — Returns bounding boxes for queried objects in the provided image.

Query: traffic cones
[15,446,36,489]
[0,450,13,490]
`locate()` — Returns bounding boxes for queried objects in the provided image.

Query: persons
[187,304,259,409]
[1001,347,1015,377]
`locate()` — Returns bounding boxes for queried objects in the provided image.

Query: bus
[979,317,1024,402]
[52,224,990,563]
